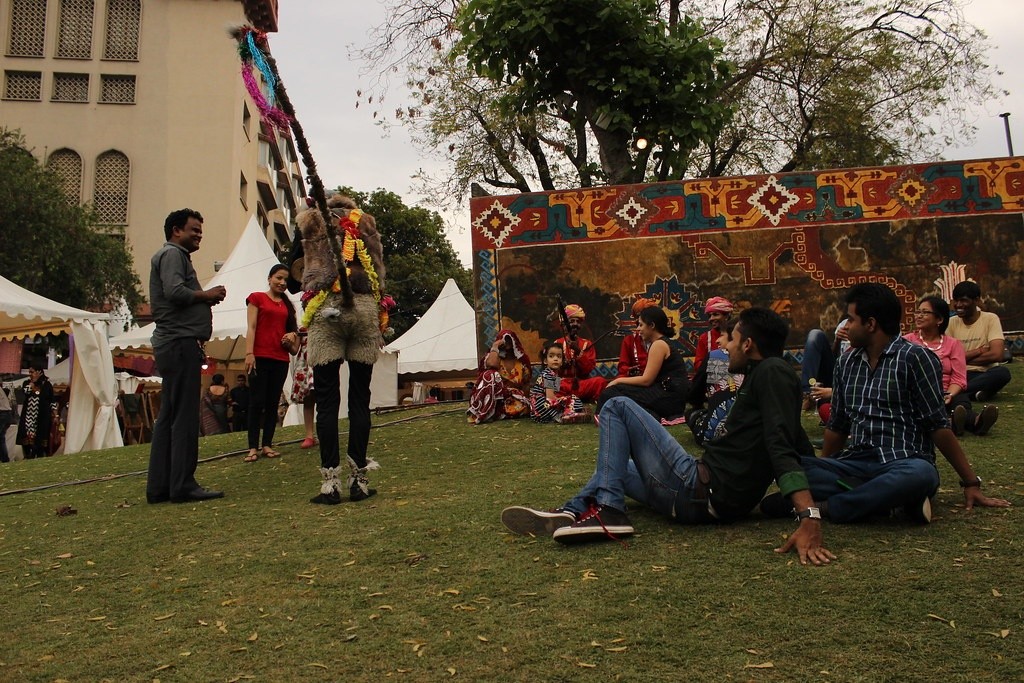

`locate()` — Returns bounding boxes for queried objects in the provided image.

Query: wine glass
[808,378,823,417]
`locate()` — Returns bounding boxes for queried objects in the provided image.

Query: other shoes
[301,438,316,448]
[951,405,965,437]
[973,404,998,436]
[760,491,795,519]
[899,495,932,525]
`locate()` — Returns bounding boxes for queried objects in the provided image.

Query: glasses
[914,310,933,316]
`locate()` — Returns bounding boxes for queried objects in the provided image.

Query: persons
[280,190,386,505]
[425,387,442,403]
[242,263,298,463]
[757,280,1013,529]
[230,374,252,432]
[147,207,226,505]
[466,296,744,448]
[207,374,231,434]
[501,308,841,567]
[14,365,59,460]
[281,299,324,449]
[0,387,14,463]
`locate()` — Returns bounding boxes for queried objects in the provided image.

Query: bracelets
[246,353,253,356]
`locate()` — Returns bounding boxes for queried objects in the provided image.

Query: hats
[704,297,733,314]
[633,297,658,319]
[559,304,586,324]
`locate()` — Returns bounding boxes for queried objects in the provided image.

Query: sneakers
[553,505,634,544]
[502,506,576,537]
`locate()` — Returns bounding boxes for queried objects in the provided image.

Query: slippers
[244,454,259,462]
[261,452,280,458]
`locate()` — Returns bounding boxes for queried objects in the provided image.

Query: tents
[105,214,310,376]
[0,274,126,453]
[378,277,479,374]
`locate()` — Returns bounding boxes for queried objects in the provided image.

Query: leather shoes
[147,495,170,504]
[170,486,223,503]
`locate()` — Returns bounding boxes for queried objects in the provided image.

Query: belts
[693,462,711,524]
